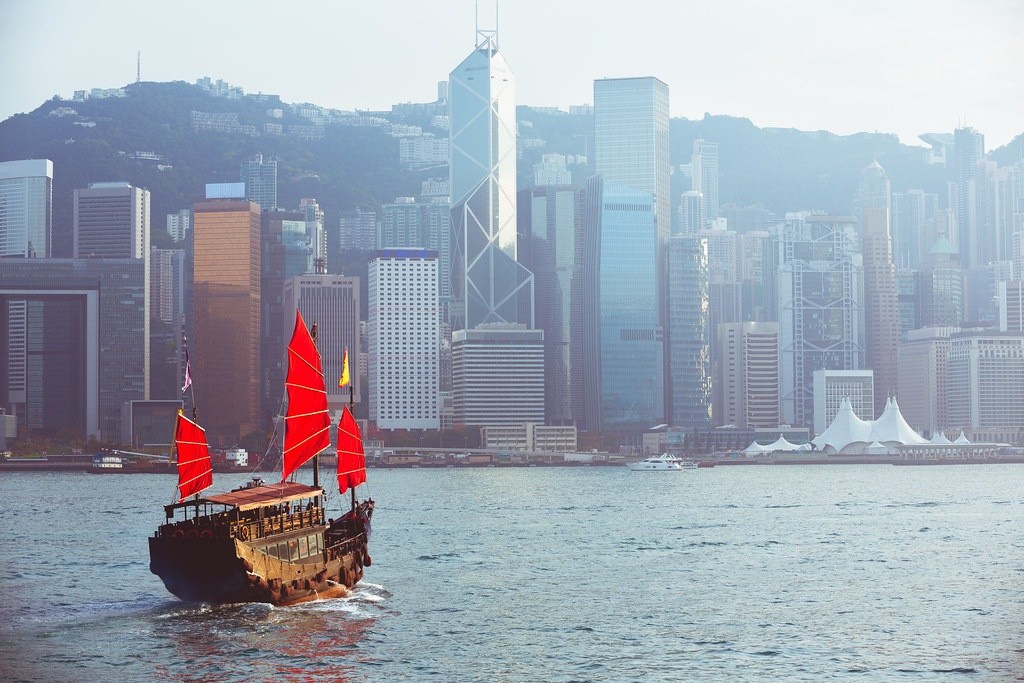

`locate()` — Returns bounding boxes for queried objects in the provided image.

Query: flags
[183,340,191,388]
[338,350,350,388]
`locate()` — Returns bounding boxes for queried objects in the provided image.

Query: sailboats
[146,304,376,608]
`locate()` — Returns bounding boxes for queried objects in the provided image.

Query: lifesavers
[186,530,200,538]
[239,527,249,540]
[200,530,214,539]
[172,529,186,538]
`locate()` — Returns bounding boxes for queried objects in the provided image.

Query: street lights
[464,436,470,449]
[420,435,424,458]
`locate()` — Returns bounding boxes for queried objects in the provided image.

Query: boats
[697,461,715,469]
[625,453,684,471]
[680,461,698,469]
[86,447,159,473]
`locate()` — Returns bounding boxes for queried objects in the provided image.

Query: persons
[283,502,290,514]
